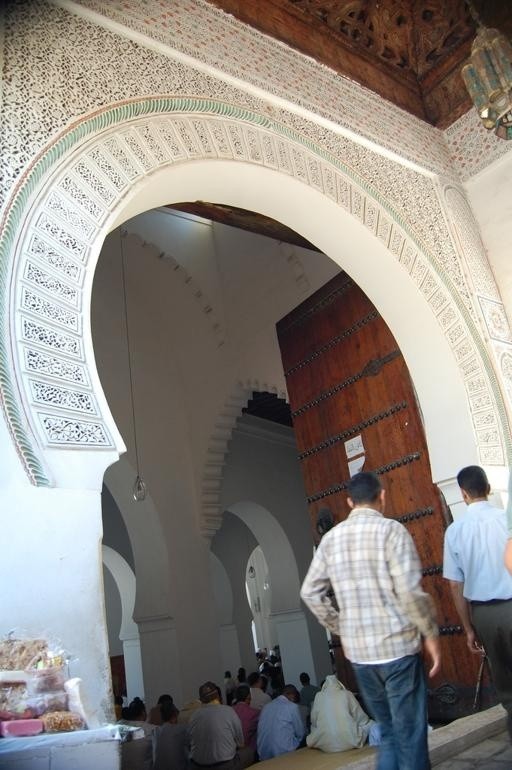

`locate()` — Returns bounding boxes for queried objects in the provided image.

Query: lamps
[119,224,147,502]
[460,0,512,141]
[245,523,256,578]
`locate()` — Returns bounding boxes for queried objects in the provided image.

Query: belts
[468,598,512,605]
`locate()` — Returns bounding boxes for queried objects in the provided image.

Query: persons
[298,472,442,769]
[116,645,375,770]
[501,471,512,580]
[443,465,512,731]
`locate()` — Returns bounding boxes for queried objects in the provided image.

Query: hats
[198,681,220,700]
